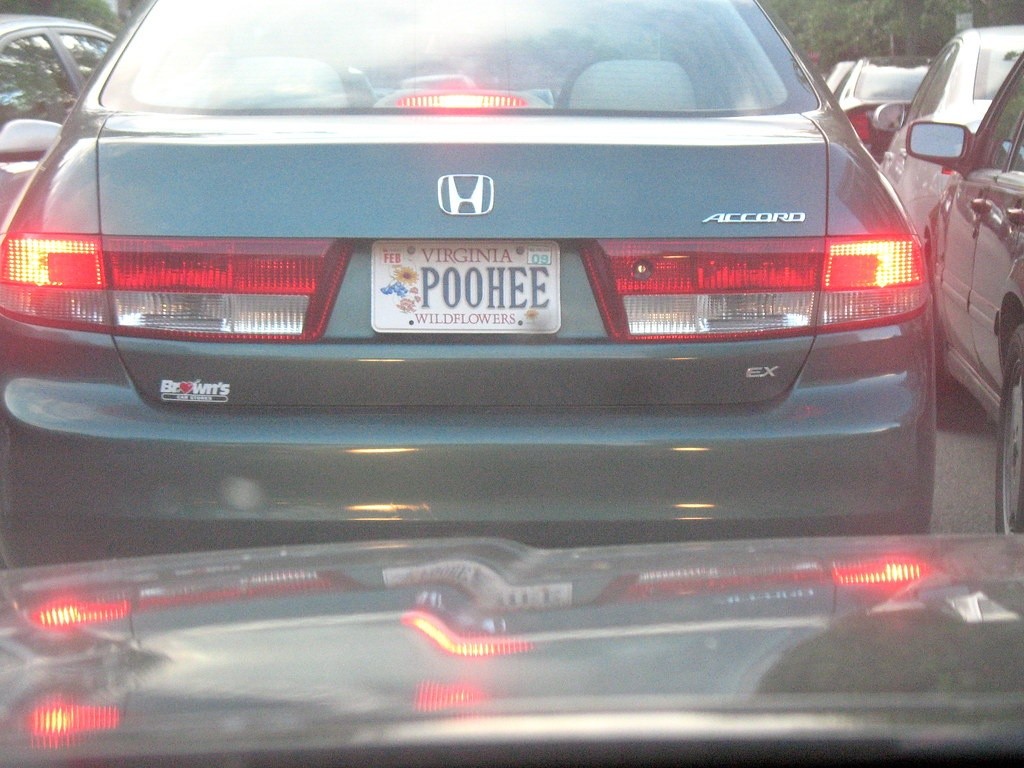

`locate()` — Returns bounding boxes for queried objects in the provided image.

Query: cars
[6,17,941,533]
[825,56,861,97]
[832,52,933,162]
[0,18,123,251]
[876,29,1024,237]
[905,47,1024,533]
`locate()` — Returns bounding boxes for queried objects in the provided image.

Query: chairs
[194,57,350,108]
[568,60,697,111]
